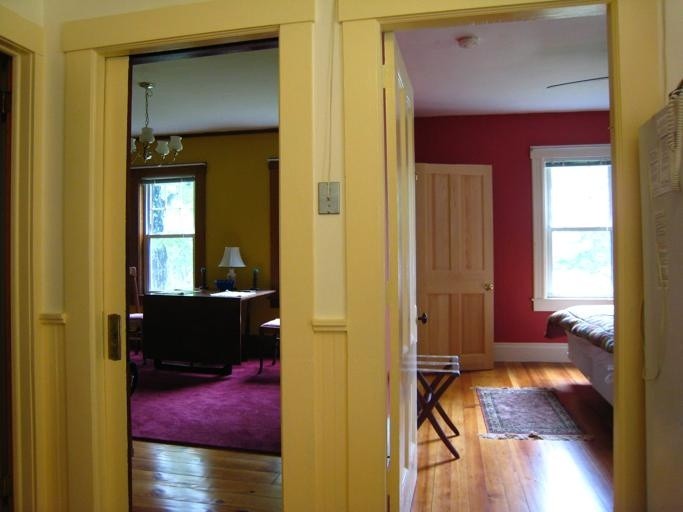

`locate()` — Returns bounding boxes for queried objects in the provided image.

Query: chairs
[258,318,280,375]
[129,268,144,355]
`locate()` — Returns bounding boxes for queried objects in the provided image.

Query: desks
[144,288,276,375]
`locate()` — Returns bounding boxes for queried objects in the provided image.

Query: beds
[543,305,614,407]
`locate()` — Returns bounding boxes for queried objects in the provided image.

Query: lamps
[130,82,183,165]
[218,247,246,287]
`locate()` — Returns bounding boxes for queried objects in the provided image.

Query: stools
[416,355,462,459]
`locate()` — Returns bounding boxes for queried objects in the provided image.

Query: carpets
[476,388,595,441]
[129,349,280,457]
